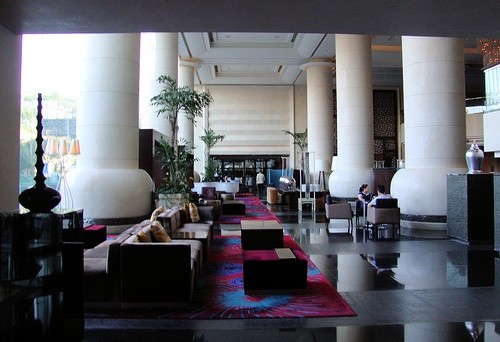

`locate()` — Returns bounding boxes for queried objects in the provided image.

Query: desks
[279,190,296,209]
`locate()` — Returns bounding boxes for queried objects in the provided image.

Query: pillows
[136,228,154,242]
[149,222,172,242]
[150,204,163,220]
[183,203,190,219]
[189,203,201,221]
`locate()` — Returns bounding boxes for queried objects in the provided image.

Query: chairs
[366,196,401,231]
[365,229,401,270]
[198,186,218,201]
[325,193,354,225]
[355,222,368,244]
[356,198,367,222]
[324,224,354,259]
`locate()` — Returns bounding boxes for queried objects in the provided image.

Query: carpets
[83,231,358,320]
[217,196,284,224]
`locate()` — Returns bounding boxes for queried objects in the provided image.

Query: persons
[357,183,397,229]
[256,170,266,200]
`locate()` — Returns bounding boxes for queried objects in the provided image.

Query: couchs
[190,192,222,218]
[156,205,176,224]
[121,220,205,310]
[84,219,153,314]
[164,203,216,249]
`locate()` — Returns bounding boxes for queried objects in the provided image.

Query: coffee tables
[83,224,107,249]
[223,199,246,215]
[240,220,283,249]
[243,247,308,297]
